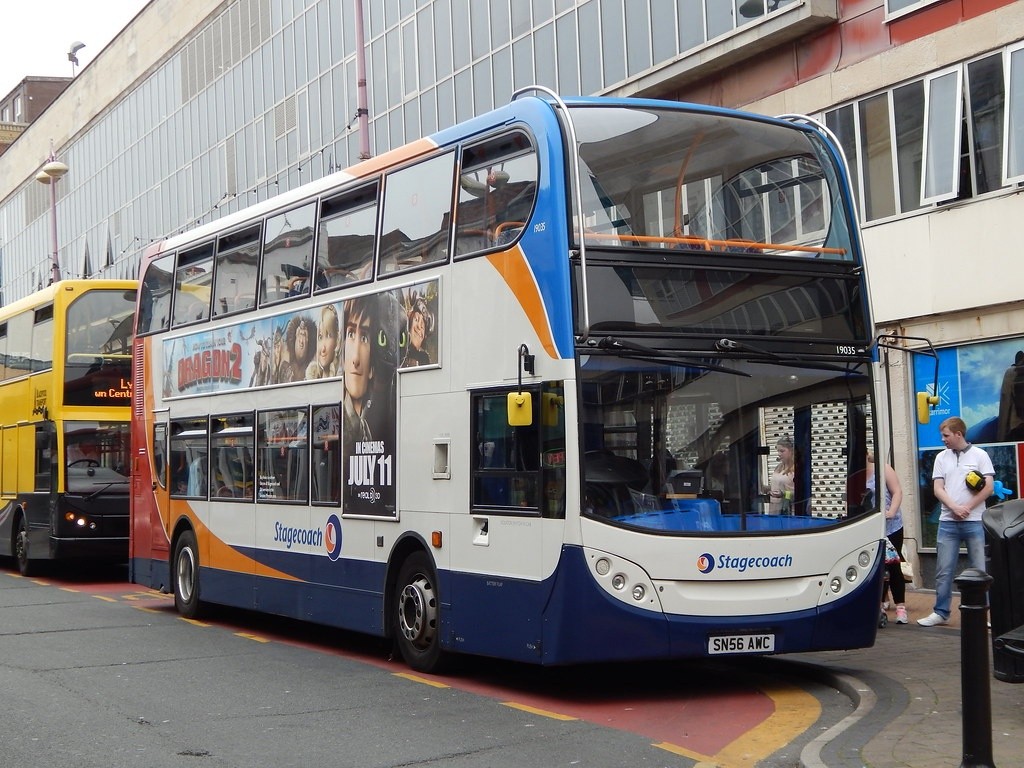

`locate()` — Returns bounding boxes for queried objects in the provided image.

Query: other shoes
[881,599,890,613]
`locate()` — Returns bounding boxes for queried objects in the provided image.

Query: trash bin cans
[981,496,1024,686]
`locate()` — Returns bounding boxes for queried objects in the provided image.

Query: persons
[170,422,186,494]
[866,446,908,624]
[761,436,794,515]
[52,434,101,468]
[916,416,996,627]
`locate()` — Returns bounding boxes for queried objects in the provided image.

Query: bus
[127,85,941,674]
[0,280,209,577]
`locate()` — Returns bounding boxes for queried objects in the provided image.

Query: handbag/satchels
[885,536,901,565]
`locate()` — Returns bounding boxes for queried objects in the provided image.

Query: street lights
[34,160,69,283]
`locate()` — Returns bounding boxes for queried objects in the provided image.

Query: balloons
[966,470,1013,499]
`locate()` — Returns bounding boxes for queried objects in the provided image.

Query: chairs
[494,222,526,246]
[316,269,359,292]
[188,301,209,320]
[574,226,602,246]
[266,286,289,303]
[457,230,493,258]
[288,276,310,297]
[669,236,715,252]
[217,484,243,498]
[720,239,763,254]
[233,292,254,311]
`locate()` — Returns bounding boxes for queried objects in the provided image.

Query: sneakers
[917,612,949,627]
[895,605,908,624]
